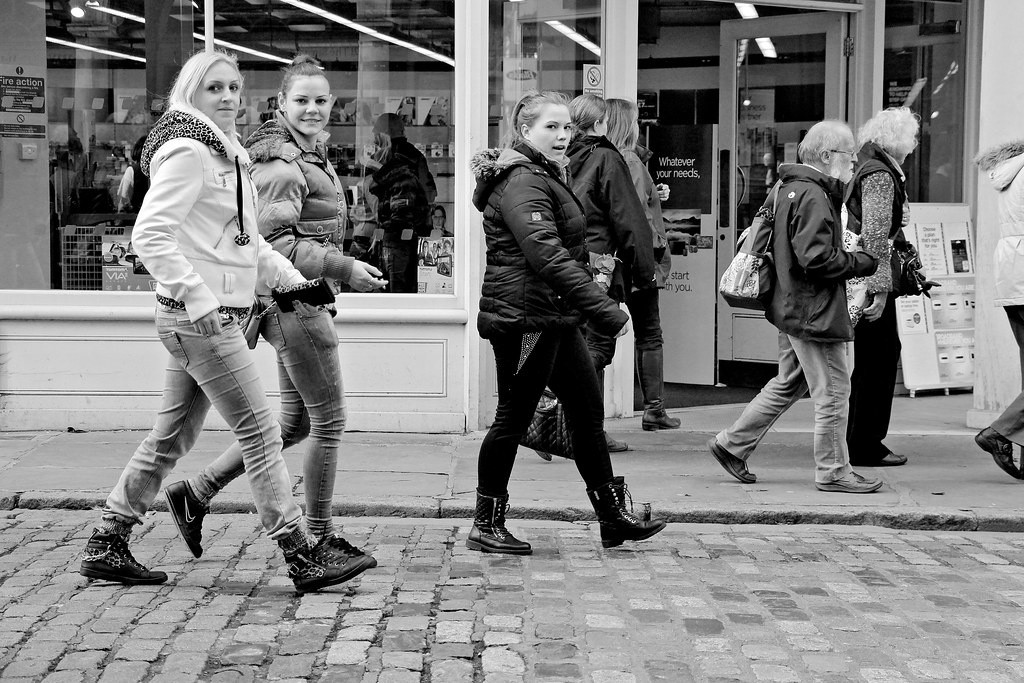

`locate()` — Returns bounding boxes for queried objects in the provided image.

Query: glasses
[827,149,854,157]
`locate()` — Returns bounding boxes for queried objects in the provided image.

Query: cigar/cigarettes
[380,278,386,289]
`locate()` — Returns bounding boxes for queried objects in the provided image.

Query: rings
[368,277,374,284]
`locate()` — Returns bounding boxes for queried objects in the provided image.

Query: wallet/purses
[271,277,335,313]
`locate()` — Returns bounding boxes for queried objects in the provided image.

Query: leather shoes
[975,427,1021,479]
[850,449,908,465]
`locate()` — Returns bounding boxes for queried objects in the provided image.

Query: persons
[365,113,438,294]
[107,243,149,276]
[534,94,656,462]
[974,130,1024,481]
[430,97,448,125]
[117,134,152,213]
[164,53,389,569]
[838,109,920,466]
[709,120,883,494]
[82,52,374,591]
[417,204,452,272]
[605,99,681,431]
[466,92,666,555]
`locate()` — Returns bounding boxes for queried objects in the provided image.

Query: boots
[586,475,666,548]
[636,347,681,430]
[598,370,628,450]
[466,488,532,554]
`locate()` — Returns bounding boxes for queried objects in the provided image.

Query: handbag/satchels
[237,294,264,349]
[517,389,574,460]
[588,252,616,293]
[898,243,942,298]
[839,159,899,325]
[718,179,784,310]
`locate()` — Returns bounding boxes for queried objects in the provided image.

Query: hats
[372,113,405,135]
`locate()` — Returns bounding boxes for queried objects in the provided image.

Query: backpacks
[374,151,430,239]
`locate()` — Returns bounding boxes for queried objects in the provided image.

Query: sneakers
[80,527,168,583]
[320,535,378,569]
[708,437,757,482]
[164,479,212,559]
[288,541,372,592]
[815,471,883,493]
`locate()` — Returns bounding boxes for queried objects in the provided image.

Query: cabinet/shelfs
[42,86,499,261]
